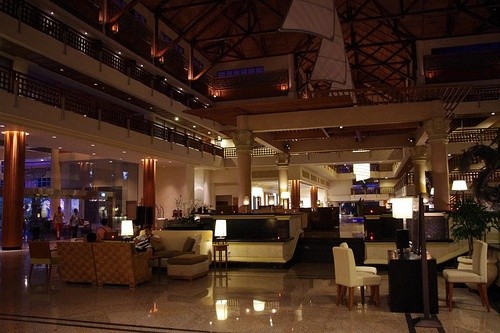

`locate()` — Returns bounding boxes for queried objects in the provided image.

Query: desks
[424,212,449,242]
[64,225,85,237]
[363,214,404,242]
[386,249,439,313]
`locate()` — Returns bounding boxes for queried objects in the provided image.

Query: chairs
[442,239,492,313]
[25,241,52,283]
[340,242,377,302]
[332,247,381,312]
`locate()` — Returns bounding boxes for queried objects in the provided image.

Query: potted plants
[442,196,500,292]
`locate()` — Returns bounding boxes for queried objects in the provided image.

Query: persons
[52,206,65,240]
[96,218,112,241]
[21,205,29,222]
[68,209,83,238]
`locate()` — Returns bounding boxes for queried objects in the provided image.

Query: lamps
[451,180,469,204]
[214,219,227,239]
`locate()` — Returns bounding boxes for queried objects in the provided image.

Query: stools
[213,245,228,271]
[215,239,227,245]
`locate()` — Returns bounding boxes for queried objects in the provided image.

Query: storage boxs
[426,204,434,212]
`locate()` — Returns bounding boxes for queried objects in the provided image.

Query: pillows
[181,237,195,253]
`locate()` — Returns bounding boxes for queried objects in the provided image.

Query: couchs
[56,240,154,288]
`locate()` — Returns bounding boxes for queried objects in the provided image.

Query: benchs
[167,254,210,282]
[139,230,212,267]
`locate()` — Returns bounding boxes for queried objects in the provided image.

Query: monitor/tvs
[120,219,134,236]
[112,216,127,231]
